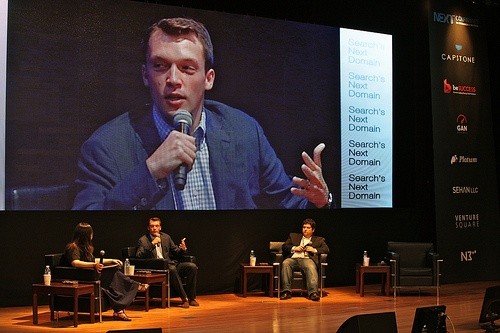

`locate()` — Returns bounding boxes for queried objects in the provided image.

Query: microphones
[99,249,105,265]
[155,233,161,246]
[172,109,192,189]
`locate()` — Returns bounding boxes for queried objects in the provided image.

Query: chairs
[125,247,171,309]
[386,241,443,296]
[268,241,328,298]
[45,253,112,321]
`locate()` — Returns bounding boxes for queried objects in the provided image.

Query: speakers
[336,310,400,333]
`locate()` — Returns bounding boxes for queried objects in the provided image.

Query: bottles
[45,266,51,275]
[363,251,367,266]
[124,258,130,275]
[250,250,254,257]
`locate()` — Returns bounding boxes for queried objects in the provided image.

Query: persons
[69,16,332,209]
[135,217,199,308]
[62,222,149,321]
[280,218,329,300]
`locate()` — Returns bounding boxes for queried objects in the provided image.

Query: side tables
[359,264,390,297]
[31,281,95,328]
[126,273,167,310]
[239,263,274,298]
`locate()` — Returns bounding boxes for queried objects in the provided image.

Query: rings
[304,180,310,188]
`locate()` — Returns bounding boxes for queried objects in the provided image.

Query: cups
[250,257,256,266]
[43,274,51,286]
[364,258,370,266]
[128,266,135,275]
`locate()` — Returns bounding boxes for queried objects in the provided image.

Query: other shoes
[181,301,190,308]
[309,292,320,301]
[280,291,291,300]
[137,283,149,293]
[190,299,199,306]
[113,311,132,321]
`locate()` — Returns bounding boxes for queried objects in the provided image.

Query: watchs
[323,193,332,208]
